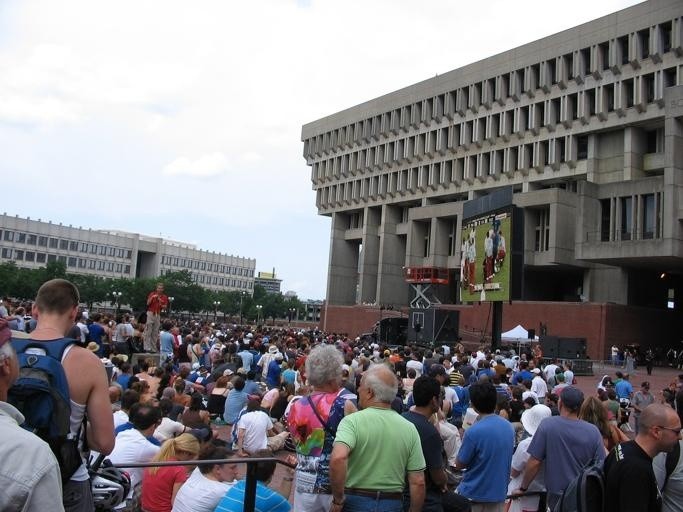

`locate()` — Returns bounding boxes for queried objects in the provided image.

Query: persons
[456,381,516,512]
[469,352,479,370]
[624,352,635,373]
[479,374,489,385]
[529,360,541,374]
[483,228,506,282]
[494,359,507,381]
[646,349,654,375]
[543,393,550,407]
[660,381,676,409]
[479,360,496,378]
[486,354,496,367]
[549,394,560,416]
[503,404,551,512]
[460,226,477,302]
[0,279,454,507]
[495,352,504,361]
[543,356,561,383]
[517,376,528,391]
[633,382,653,436]
[522,380,539,404]
[579,396,630,451]
[509,387,524,422]
[616,373,634,399]
[492,375,511,401]
[512,361,532,385]
[476,360,484,375]
[495,393,509,419]
[477,346,485,360]
[611,345,619,368]
[602,388,621,423]
[512,386,606,512]
[596,375,611,390]
[613,372,622,385]
[499,374,512,395]
[652,432,683,512]
[530,368,548,403]
[622,350,627,368]
[564,363,573,385]
[504,368,512,383]
[534,345,542,364]
[603,402,683,511]
[502,353,514,369]
[548,368,561,388]
[675,374,683,429]
[519,397,535,441]
[520,354,528,362]
[512,357,519,375]
[552,374,569,396]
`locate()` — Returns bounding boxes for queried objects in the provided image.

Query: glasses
[658,425,680,435]
[645,387,649,389]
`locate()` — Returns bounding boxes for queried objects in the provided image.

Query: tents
[500,323,539,355]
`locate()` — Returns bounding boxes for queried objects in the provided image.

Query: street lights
[168,297,174,311]
[256,305,262,322]
[213,301,220,316]
[289,308,295,321]
[239,291,246,316]
[113,292,122,315]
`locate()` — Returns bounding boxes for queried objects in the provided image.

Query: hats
[115,353,128,362]
[556,373,564,380]
[275,353,282,360]
[222,368,233,377]
[237,367,246,375]
[160,387,174,399]
[87,342,98,351]
[641,381,649,387]
[0,317,11,348]
[268,345,278,352]
[245,332,252,338]
[522,404,551,435]
[530,367,540,373]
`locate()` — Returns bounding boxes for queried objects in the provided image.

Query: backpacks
[552,463,610,512]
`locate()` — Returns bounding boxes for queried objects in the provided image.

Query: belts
[343,487,403,499]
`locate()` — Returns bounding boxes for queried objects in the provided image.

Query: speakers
[526,328,535,339]
[414,323,422,333]
